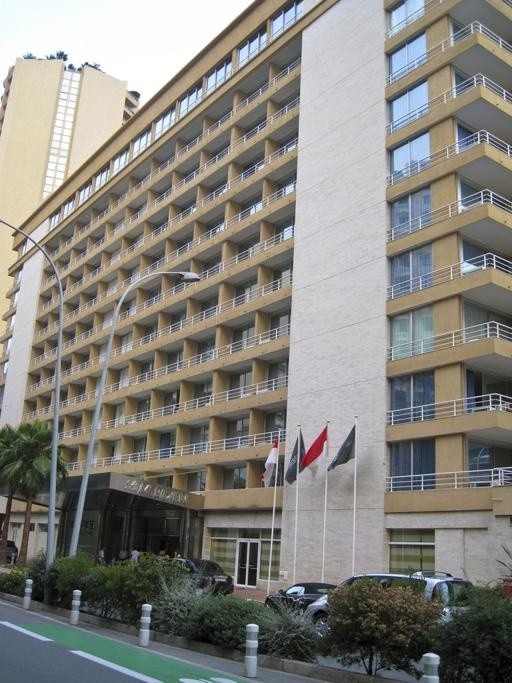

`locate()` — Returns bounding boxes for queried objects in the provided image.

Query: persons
[174,551,182,557]
[118,544,144,563]
[97,545,107,567]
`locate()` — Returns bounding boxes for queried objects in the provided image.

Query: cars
[169,559,234,597]
[264,583,338,618]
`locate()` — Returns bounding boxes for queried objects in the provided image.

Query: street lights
[68,271,201,559]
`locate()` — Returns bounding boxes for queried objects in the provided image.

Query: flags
[327,424,355,473]
[284,432,307,484]
[300,426,332,477]
[261,432,278,489]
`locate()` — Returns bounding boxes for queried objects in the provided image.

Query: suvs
[302,570,477,660]
[0,541,18,564]
